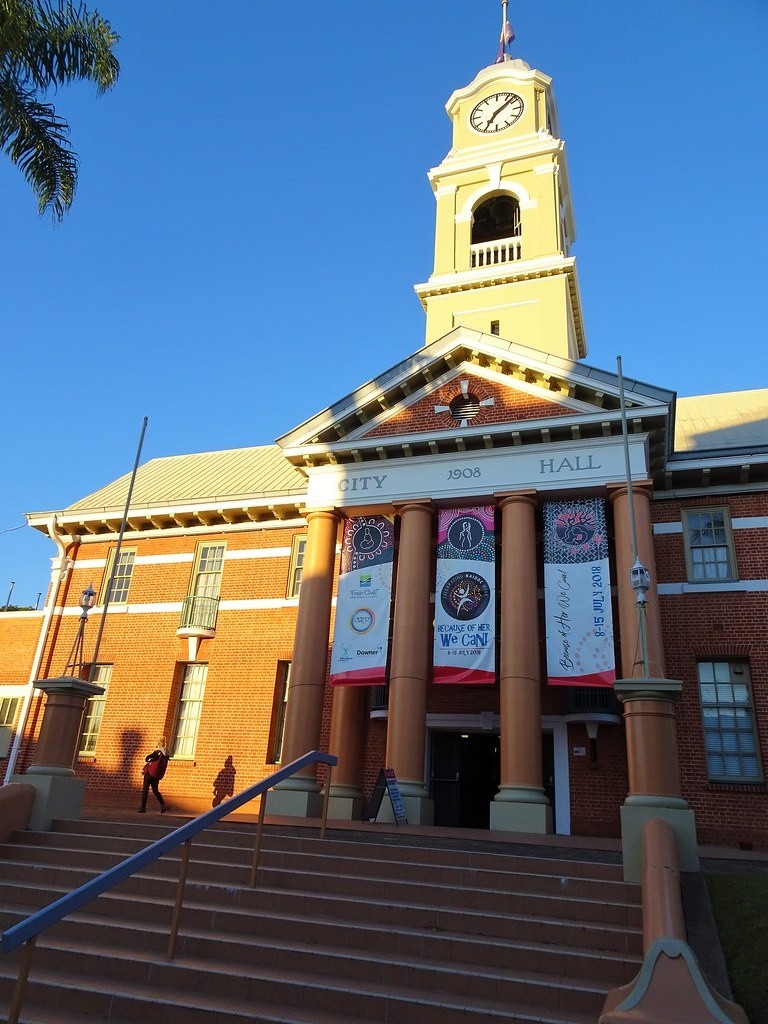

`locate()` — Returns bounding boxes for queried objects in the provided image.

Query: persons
[137,737,170,814]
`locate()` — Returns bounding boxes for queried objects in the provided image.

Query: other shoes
[137,809,146,813]
[160,808,166,814]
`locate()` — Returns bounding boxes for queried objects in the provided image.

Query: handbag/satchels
[146,750,161,762]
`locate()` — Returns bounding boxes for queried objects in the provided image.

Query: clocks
[471,93,523,134]
